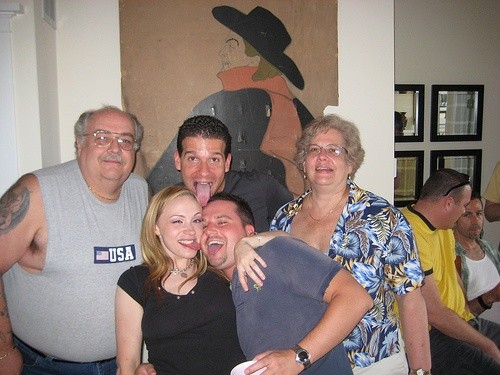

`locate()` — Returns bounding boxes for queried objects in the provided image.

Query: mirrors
[394,150,424,207]
[430,149,482,199]
[430,84,484,142]
[394,83,424,142]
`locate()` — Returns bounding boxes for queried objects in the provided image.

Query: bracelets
[478,294,493,310]
[1,345,16,362]
[249,230,261,247]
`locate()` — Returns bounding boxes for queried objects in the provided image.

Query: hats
[212,4,305,90]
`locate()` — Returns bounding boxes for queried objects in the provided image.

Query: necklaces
[85,181,120,199]
[305,184,350,221]
[168,259,196,280]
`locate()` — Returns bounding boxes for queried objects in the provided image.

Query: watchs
[291,344,311,370]
[409,368,431,374]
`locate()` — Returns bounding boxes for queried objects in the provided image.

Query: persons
[482,160,500,255]
[0,104,154,375]
[169,116,298,235]
[389,167,500,375]
[113,181,291,375]
[135,192,374,374]
[270,114,433,374]
[452,192,500,326]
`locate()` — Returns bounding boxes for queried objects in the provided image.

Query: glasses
[81,129,137,151]
[443,173,472,196]
[305,144,348,157]
